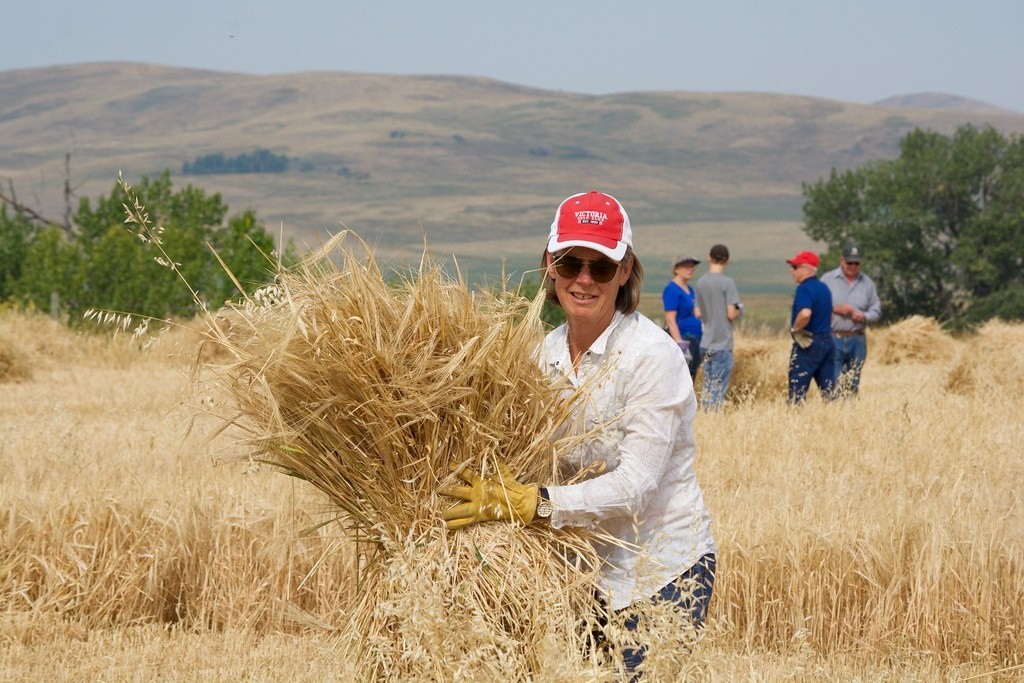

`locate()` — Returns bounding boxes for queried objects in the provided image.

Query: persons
[784,250,838,407]
[821,241,883,402]
[432,190,718,683]
[656,243,747,417]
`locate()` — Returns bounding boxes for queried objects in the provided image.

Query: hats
[787,251,820,267]
[546,190,633,261]
[710,245,729,261]
[673,253,701,267]
[843,242,862,263]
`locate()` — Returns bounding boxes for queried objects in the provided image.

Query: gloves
[436,457,537,530]
[791,329,813,349]
[678,341,693,364]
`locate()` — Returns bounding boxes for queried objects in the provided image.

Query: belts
[832,331,863,337]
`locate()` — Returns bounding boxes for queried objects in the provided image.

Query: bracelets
[863,314,867,326]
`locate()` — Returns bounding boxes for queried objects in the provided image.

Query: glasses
[847,261,860,266]
[552,252,622,283]
[791,264,808,270]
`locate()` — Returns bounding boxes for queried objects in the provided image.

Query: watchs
[790,327,795,334]
[536,486,554,522]
